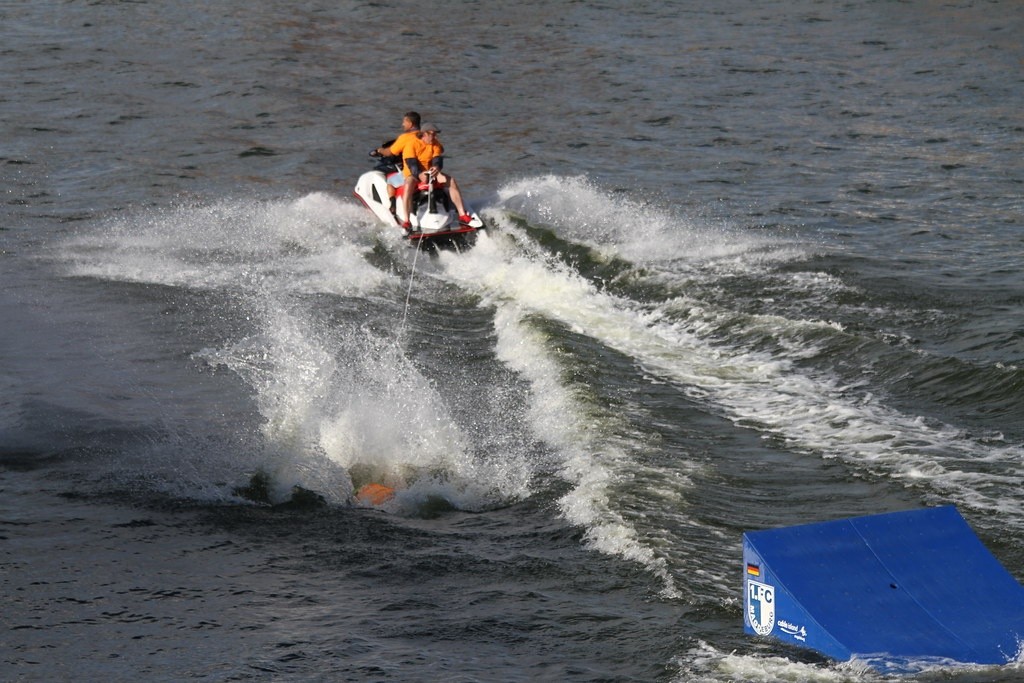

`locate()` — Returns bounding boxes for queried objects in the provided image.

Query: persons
[403,123,472,232]
[376,111,445,214]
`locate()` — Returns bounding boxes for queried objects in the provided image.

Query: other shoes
[404,221,413,233]
[459,214,473,223]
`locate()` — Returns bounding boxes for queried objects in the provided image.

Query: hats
[421,123,441,134]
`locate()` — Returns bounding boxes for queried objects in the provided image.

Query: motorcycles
[353,138,486,248]
